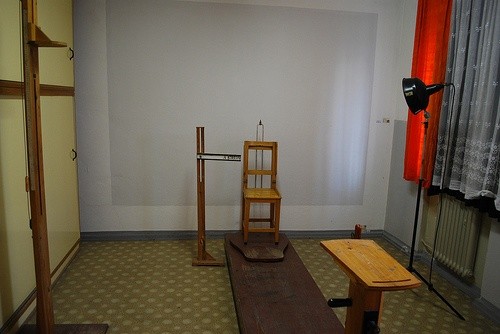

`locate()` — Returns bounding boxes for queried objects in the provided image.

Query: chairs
[240,141,282,244]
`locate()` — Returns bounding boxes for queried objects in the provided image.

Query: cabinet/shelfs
[0,0,80,334]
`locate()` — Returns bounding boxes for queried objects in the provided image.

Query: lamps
[402,77,468,321]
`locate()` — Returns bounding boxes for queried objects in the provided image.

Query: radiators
[433,193,483,280]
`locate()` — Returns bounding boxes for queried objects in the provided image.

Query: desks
[320,239,421,334]
